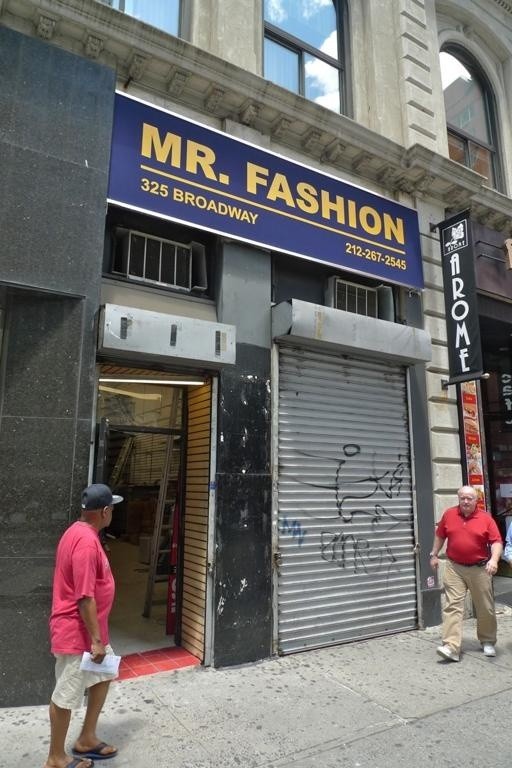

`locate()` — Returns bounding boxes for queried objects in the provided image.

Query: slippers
[66,757,94,768]
[72,742,116,759]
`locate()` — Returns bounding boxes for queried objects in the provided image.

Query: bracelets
[429,553,437,559]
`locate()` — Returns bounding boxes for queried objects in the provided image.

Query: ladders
[108,437,134,492]
[141,386,180,620]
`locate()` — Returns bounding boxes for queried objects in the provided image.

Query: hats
[81,484,124,510]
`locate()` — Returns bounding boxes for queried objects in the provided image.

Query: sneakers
[436,647,460,662]
[482,642,496,656]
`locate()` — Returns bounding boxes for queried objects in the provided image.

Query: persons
[43,484,124,768]
[429,486,503,661]
[504,520,512,568]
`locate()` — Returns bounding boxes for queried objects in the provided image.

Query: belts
[455,561,479,566]
